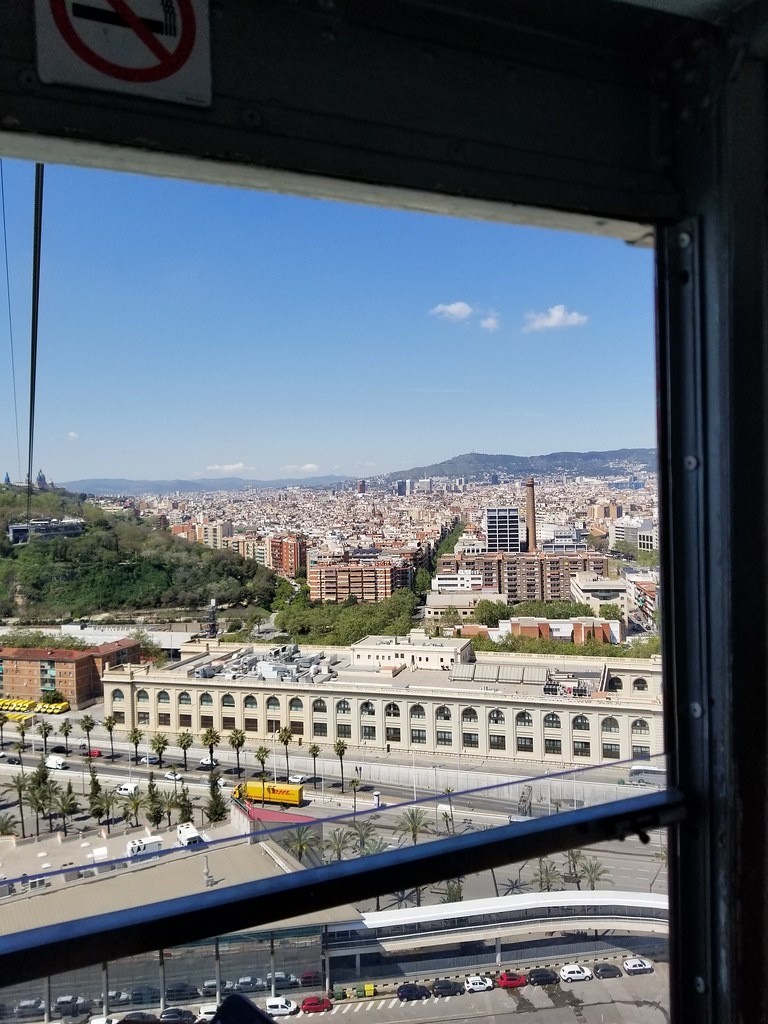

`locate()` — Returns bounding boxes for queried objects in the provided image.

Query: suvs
[16,999,45,1018]
[166,983,200,1000]
[54,996,87,1010]
[202,979,233,996]
[624,958,654,976]
[131,986,162,1004]
[100,991,129,1005]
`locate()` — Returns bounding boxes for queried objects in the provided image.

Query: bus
[630,766,667,785]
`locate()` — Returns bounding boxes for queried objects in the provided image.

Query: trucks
[44,756,68,770]
[232,782,304,807]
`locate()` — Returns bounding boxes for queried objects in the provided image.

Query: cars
[199,758,218,765]
[594,964,622,979]
[528,969,560,986]
[397,984,432,1002]
[301,997,333,1015]
[464,976,495,993]
[433,979,464,999]
[7,757,21,764]
[165,771,182,780]
[87,750,101,757]
[300,971,324,987]
[50,746,65,753]
[497,973,527,989]
[560,965,593,983]
[233,976,265,992]
[291,774,305,784]
[140,757,159,764]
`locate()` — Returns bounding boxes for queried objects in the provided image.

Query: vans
[116,783,139,796]
[267,972,299,989]
[6,713,38,724]
[160,1008,194,1023]
[0,699,37,711]
[34,702,70,714]
[198,1005,219,1024]
[266,998,299,1017]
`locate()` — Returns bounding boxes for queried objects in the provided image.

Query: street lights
[270,730,280,785]
[433,764,443,834]
[243,749,251,783]
[76,735,86,797]
[122,733,133,783]
[140,717,154,766]
